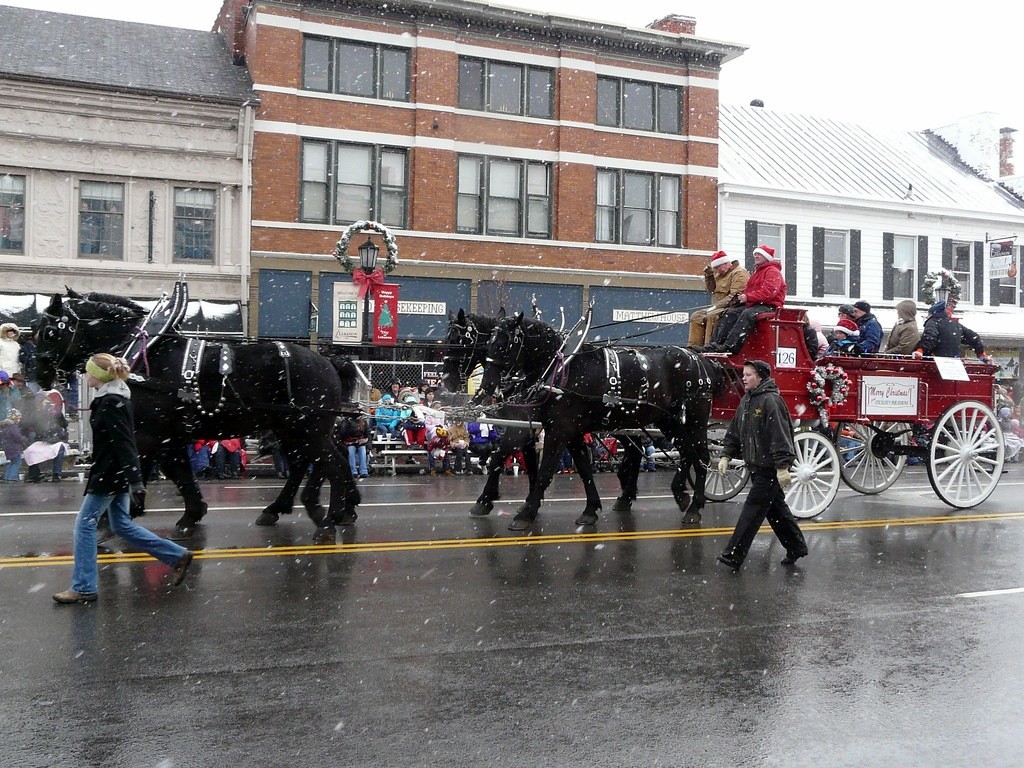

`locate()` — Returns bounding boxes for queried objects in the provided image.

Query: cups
[513,466,519,476]
[459,440,464,449]
[78,473,85,481]
[19,474,25,480]
[385,433,391,441]
[377,434,383,441]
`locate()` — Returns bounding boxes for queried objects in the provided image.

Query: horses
[25,285,366,538]
[438,306,735,530]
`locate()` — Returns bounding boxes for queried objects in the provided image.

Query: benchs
[758,308,808,322]
[73,431,496,478]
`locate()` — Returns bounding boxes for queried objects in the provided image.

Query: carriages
[19,279,1006,543]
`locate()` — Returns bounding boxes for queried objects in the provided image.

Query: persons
[716,361,808,568]
[0,322,69,483]
[809,380,1024,467]
[912,299,995,366]
[709,244,787,353]
[685,251,751,352]
[884,301,921,354]
[188,438,313,479]
[52,352,192,604]
[803,300,884,362]
[338,376,656,478]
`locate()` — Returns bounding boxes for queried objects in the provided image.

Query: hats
[834,319,860,336]
[9,373,26,385]
[929,300,945,315]
[389,376,401,389]
[745,360,771,381]
[839,302,870,318]
[8,408,22,419]
[711,251,730,267]
[753,245,774,261]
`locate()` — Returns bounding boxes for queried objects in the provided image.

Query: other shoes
[716,553,739,571]
[8,480,24,484]
[53,472,61,482]
[781,552,808,564]
[688,342,728,352]
[353,465,488,480]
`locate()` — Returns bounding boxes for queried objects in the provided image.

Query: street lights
[358,236,381,372]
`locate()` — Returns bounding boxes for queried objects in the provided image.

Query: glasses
[838,313,844,316]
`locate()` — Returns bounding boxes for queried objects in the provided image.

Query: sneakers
[53,588,98,603]
[174,551,193,586]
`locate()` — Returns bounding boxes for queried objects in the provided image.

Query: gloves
[854,346,864,355]
[978,352,993,362]
[776,468,791,488]
[718,456,730,476]
[912,347,924,359]
[130,480,146,507]
[827,335,834,345]
